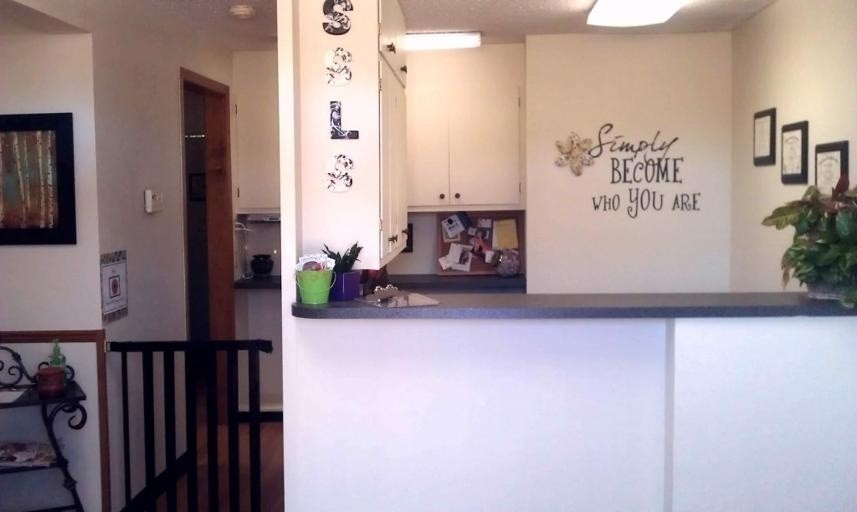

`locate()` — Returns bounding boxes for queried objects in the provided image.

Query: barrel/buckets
[295,269,337,305]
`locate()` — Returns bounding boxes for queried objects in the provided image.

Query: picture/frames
[753,108,848,196]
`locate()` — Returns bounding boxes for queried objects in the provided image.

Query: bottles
[49,338,66,370]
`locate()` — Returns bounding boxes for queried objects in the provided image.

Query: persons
[473,229,486,254]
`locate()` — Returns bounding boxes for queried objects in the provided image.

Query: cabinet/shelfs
[296,0,406,271]
[405,42,525,213]
[0,383,87,511]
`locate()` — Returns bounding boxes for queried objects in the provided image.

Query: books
[1,442,57,468]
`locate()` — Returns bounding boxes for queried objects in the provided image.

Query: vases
[38,368,65,397]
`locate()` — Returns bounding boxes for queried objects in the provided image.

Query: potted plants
[321,244,361,303]
[763,173,857,310]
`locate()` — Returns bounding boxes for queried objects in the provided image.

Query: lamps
[404,31,481,50]
[586,0,686,27]
